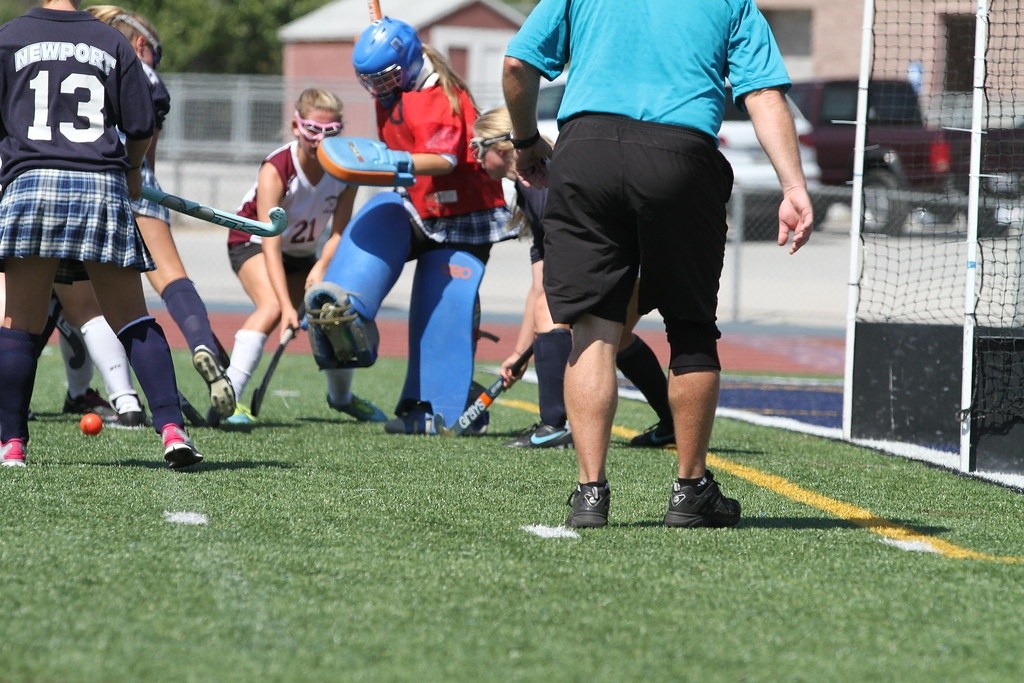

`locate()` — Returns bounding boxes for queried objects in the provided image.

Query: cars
[497,76,826,239]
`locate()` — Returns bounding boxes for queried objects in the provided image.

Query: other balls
[80,413,102,435]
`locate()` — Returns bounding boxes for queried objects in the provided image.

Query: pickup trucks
[784,73,1024,237]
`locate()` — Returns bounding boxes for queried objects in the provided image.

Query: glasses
[294,110,344,141]
[468,133,512,160]
[116,14,162,69]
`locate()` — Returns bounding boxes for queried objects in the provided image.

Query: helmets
[351,17,425,106]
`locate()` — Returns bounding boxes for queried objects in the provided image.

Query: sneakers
[662,469,742,527]
[219,403,251,424]
[383,408,489,434]
[567,480,611,528]
[109,390,146,427]
[320,303,357,363]
[0,438,26,469]
[63,388,119,420]
[159,422,203,468]
[326,392,387,422]
[627,415,677,447]
[503,422,574,451]
[192,346,236,417]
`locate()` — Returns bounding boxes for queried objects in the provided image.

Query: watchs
[509,129,540,148]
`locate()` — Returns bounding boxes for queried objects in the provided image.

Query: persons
[0,0,205,469]
[502,0,815,526]
[22,7,678,450]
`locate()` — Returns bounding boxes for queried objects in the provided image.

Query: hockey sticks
[54,316,86,369]
[139,185,289,237]
[250,306,305,416]
[174,388,221,428]
[434,344,536,439]
